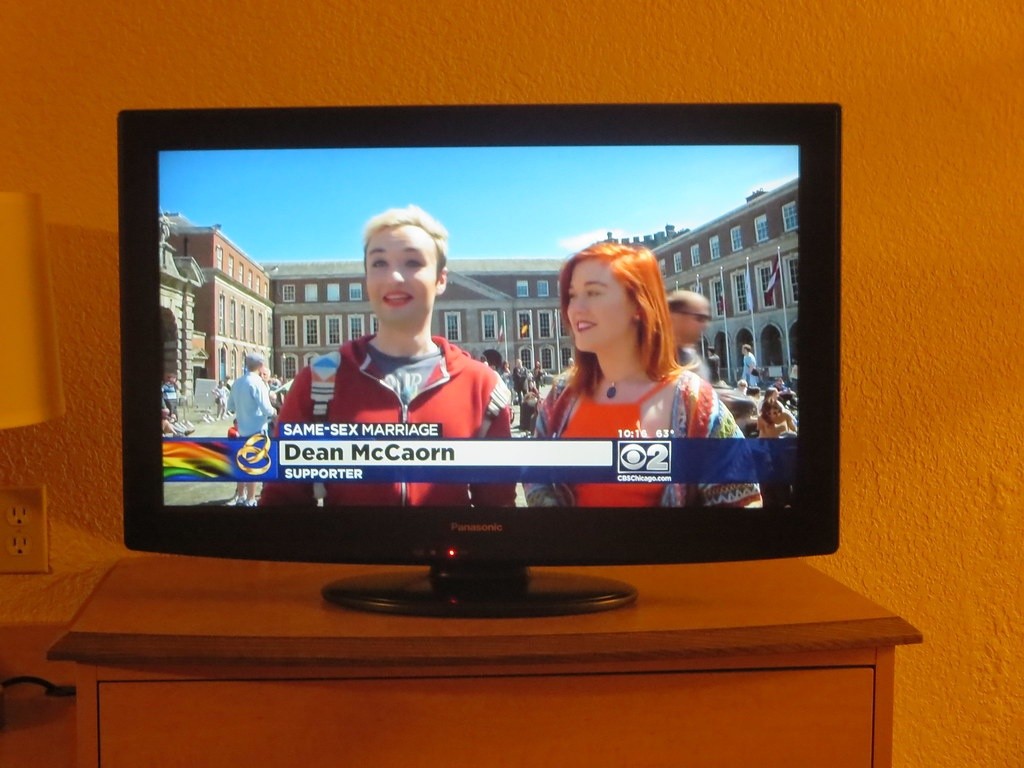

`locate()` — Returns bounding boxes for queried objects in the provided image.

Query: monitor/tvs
[116,101,844,619]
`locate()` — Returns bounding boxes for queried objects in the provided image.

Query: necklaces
[606,379,616,398]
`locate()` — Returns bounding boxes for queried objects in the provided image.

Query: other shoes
[185,429,195,436]
[225,495,238,505]
[237,497,257,506]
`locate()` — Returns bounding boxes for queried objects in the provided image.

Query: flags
[498,315,556,343]
[672,248,784,319]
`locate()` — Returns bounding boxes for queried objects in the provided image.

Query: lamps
[0,191,63,430]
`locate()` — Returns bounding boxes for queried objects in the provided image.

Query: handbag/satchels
[752,370,760,376]
[215,398,219,404]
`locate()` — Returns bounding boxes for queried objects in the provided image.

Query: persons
[665,290,714,388]
[523,239,765,513]
[159,210,171,243]
[210,354,287,508]
[480,354,575,436]
[257,203,518,508]
[161,372,195,437]
[664,344,799,439]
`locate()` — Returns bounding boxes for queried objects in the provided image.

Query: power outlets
[0,484,48,573]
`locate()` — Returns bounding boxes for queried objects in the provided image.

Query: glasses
[680,312,712,322]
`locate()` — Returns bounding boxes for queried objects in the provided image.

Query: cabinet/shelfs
[48,556,924,768]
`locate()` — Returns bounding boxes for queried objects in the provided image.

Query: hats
[246,354,264,366]
